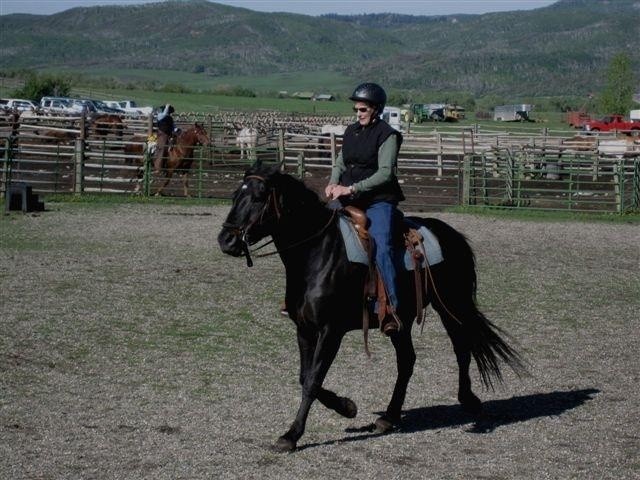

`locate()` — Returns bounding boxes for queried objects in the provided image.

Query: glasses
[352,108,367,112]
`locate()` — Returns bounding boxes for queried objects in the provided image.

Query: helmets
[349,82,386,110]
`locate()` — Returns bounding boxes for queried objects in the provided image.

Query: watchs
[349,185,354,195]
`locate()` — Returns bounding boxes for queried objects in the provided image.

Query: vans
[377,106,404,133]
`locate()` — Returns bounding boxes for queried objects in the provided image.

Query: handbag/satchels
[337,165,374,205]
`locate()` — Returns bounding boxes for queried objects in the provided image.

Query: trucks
[567,111,591,128]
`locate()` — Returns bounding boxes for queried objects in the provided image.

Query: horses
[90,114,128,149]
[1,125,93,192]
[217,155,534,455]
[124,120,212,199]
[318,134,344,167]
[559,135,596,158]
[234,127,258,161]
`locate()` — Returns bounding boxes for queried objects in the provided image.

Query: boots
[384,322,398,333]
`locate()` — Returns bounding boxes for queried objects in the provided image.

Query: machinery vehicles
[424,101,465,122]
[400,103,428,123]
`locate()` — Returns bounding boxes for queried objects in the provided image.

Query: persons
[154,105,175,173]
[326,83,407,334]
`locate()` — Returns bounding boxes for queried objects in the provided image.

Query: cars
[581,115,640,137]
[0,96,154,120]
